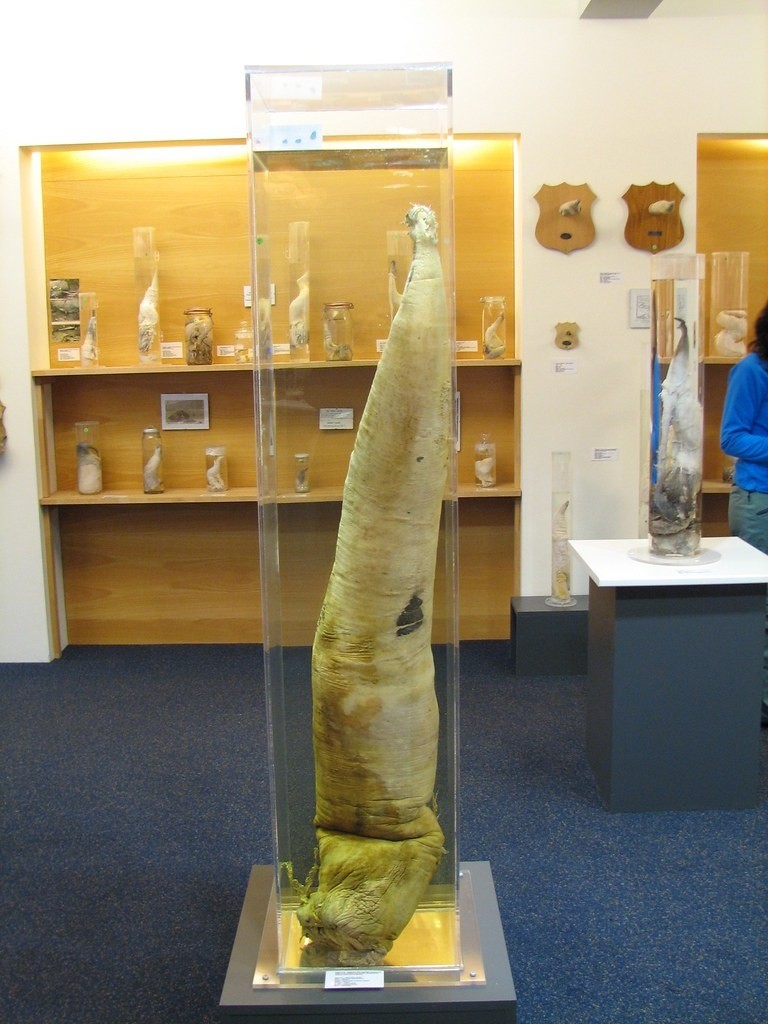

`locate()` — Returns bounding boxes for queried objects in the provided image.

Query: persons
[720,300,768,726]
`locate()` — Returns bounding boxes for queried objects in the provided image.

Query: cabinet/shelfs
[650,356,745,493]
[33,359,521,506]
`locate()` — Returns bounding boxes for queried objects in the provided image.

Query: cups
[709,252,750,357]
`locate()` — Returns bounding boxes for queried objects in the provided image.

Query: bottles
[142,424,164,493]
[322,302,356,360]
[75,420,102,495]
[206,446,228,493]
[474,433,496,488]
[184,309,213,365]
[294,454,309,493]
[481,297,506,360]
[234,321,254,363]
